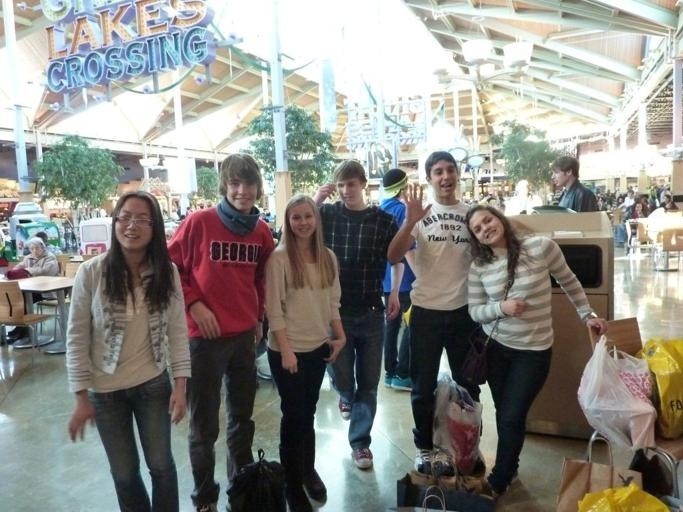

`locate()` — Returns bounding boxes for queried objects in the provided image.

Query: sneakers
[415,447,433,471]
[390,374,412,391]
[338,397,352,419]
[352,447,373,469]
[384,371,397,387]
[196,501,217,512]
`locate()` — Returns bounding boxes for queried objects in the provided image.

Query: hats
[381,168,408,200]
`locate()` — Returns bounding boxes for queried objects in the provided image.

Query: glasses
[114,215,153,227]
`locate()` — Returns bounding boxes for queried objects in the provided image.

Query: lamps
[462,16,534,83]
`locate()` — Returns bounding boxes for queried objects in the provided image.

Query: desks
[0,276,73,355]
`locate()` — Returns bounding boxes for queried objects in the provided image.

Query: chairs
[35,255,70,341]
[585,317,683,499]
[626,208,683,271]
[0,282,50,367]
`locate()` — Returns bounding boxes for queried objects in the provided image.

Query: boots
[8,327,21,337]
[15,327,29,341]
[303,441,327,503]
[278,444,313,511]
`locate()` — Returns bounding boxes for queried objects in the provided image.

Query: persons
[277,160,404,469]
[5,179,679,339]
[378,169,418,391]
[387,151,487,476]
[68,192,192,511]
[166,154,275,511]
[549,156,600,213]
[465,206,608,499]
[264,195,346,511]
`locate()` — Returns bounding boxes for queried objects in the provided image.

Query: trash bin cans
[504,211,614,440]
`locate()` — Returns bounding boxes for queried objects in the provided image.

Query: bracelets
[257,318,263,322]
[582,311,597,323]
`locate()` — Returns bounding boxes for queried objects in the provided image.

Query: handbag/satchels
[453,332,489,385]
[387,450,497,512]
[4,268,31,280]
[0,250,8,266]
[555,339,683,511]
